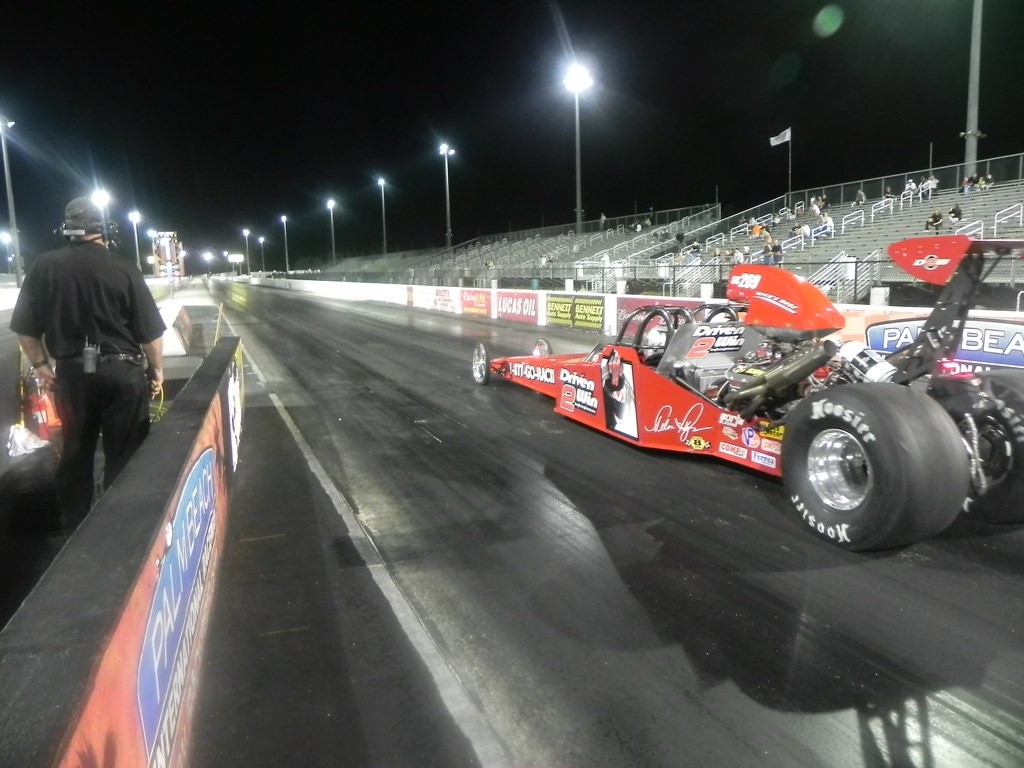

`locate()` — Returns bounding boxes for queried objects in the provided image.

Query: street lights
[378,177,388,261]
[129,211,144,278]
[0,114,24,288]
[93,190,109,248]
[439,144,455,258]
[566,67,585,237]
[145,228,158,271]
[281,214,290,275]
[259,237,266,274]
[243,227,251,276]
[328,199,337,269]
[0,232,11,278]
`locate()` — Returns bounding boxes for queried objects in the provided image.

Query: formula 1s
[469,231,1023,556]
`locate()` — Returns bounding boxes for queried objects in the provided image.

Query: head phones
[58,222,119,245]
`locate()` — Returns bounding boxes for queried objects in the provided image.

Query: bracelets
[33,360,48,368]
[151,367,163,373]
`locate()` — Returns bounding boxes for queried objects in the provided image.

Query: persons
[948,204,961,231]
[901,174,939,199]
[529,254,553,277]
[925,207,943,235]
[651,227,670,244]
[486,259,494,268]
[599,213,607,230]
[9,196,167,534]
[676,195,834,266]
[402,253,406,259]
[628,207,656,233]
[850,189,866,206]
[881,185,896,205]
[959,172,994,195]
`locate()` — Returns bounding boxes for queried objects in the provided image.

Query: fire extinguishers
[28,392,49,441]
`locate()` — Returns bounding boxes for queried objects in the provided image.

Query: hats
[65,198,102,233]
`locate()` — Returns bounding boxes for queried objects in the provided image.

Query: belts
[100,353,143,361]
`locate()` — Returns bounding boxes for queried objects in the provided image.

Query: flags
[770,128,791,147]
